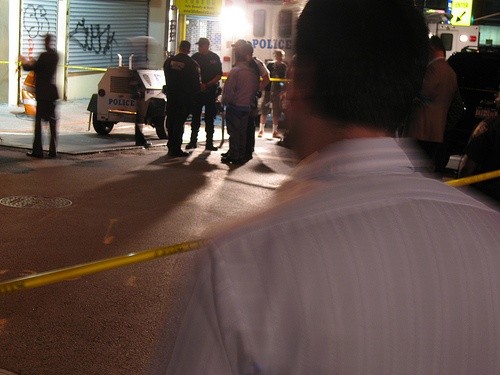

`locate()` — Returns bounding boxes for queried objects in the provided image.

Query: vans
[217,0,307,122]
[429,23,481,60]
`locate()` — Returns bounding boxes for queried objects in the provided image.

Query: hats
[231,40,248,47]
[273,49,285,56]
[177,40,191,48]
[195,38,209,44]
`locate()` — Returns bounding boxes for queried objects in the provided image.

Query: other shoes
[26,151,44,158]
[205,144,218,151]
[272,132,282,139]
[44,153,57,159]
[186,143,197,149]
[167,151,189,157]
[258,131,264,137]
[221,150,253,162]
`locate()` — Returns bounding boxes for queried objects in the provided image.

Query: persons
[18,33,60,158]
[157,33,299,164]
[162,1,500,375]
[422,31,500,205]
